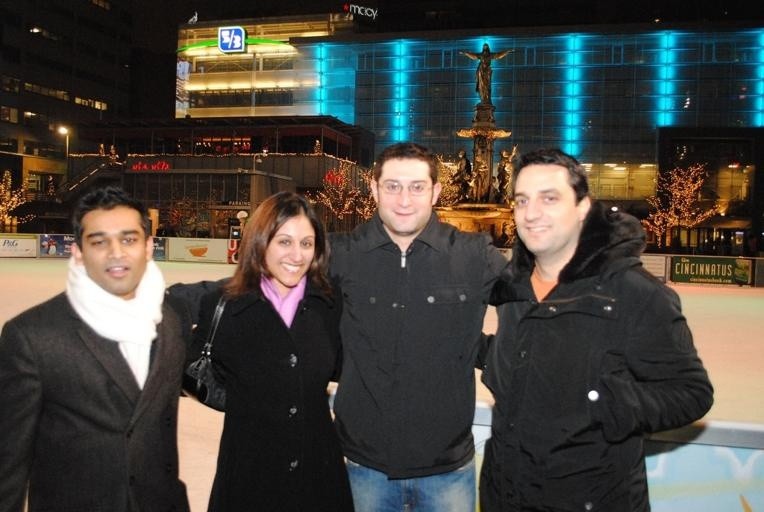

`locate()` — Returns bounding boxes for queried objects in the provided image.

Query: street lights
[57,126,71,158]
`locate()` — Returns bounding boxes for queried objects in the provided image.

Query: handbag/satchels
[178,273,245,416]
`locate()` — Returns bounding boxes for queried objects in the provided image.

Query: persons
[318,142,511,512]
[480,145,715,512]
[0,184,198,512]
[177,188,495,512]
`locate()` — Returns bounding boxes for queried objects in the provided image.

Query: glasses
[376,178,434,195]
